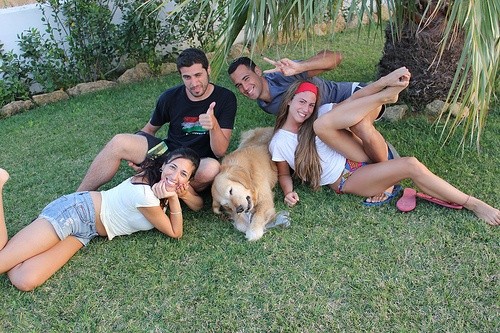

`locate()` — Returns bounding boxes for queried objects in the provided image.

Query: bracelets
[170,209,183,215]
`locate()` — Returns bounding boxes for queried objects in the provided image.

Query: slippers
[396,188,416,212]
[416,192,464,209]
[364,184,402,206]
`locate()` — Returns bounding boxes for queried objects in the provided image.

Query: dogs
[211,127,279,242]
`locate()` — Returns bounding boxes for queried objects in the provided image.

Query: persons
[0,150,204,292]
[77,48,237,192]
[267,65,500,225]
[228,50,403,206]
